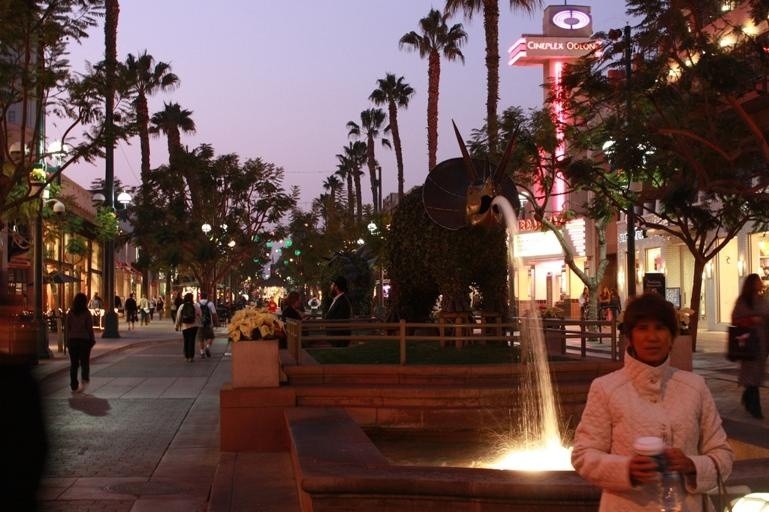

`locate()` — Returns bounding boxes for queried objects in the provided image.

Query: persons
[608,289,622,319]
[239,295,247,310]
[306,294,320,320]
[727,272,769,423]
[63,291,96,394]
[255,297,265,308]
[87,291,164,332]
[571,292,734,512]
[579,287,590,328]
[324,275,354,348]
[267,295,277,312]
[194,291,219,361]
[599,285,610,328]
[173,292,201,362]
[216,295,232,323]
[280,290,309,349]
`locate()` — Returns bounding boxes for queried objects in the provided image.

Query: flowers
[538,304,565,328]
[226,304,285,342]
[672,305,695,335]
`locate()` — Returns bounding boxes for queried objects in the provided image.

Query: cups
[633,436,666,484]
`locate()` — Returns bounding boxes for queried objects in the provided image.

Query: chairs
[17,307,123,332]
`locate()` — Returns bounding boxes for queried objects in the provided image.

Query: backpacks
[198,300,211,326]
[181,302,195,323]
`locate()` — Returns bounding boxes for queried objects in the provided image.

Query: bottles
[659,469,682,512]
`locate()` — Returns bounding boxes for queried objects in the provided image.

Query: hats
[330,275,347,288]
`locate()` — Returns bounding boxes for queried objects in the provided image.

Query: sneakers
[185,348,211,362]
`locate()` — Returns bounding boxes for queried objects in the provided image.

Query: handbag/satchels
[728,326,758,362]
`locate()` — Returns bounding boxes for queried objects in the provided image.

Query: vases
[670,335,692,371]
[230,337,280,387]
[543,328,562,361]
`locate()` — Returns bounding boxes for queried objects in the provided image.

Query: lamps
[738,259,744,277]
[706,261,712,279]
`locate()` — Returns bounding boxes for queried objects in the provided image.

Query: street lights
[200,222,237,305]
[8,138,69,361]
[90,189,135,339]
[366,218,392,311]
[602,137,655,304]
